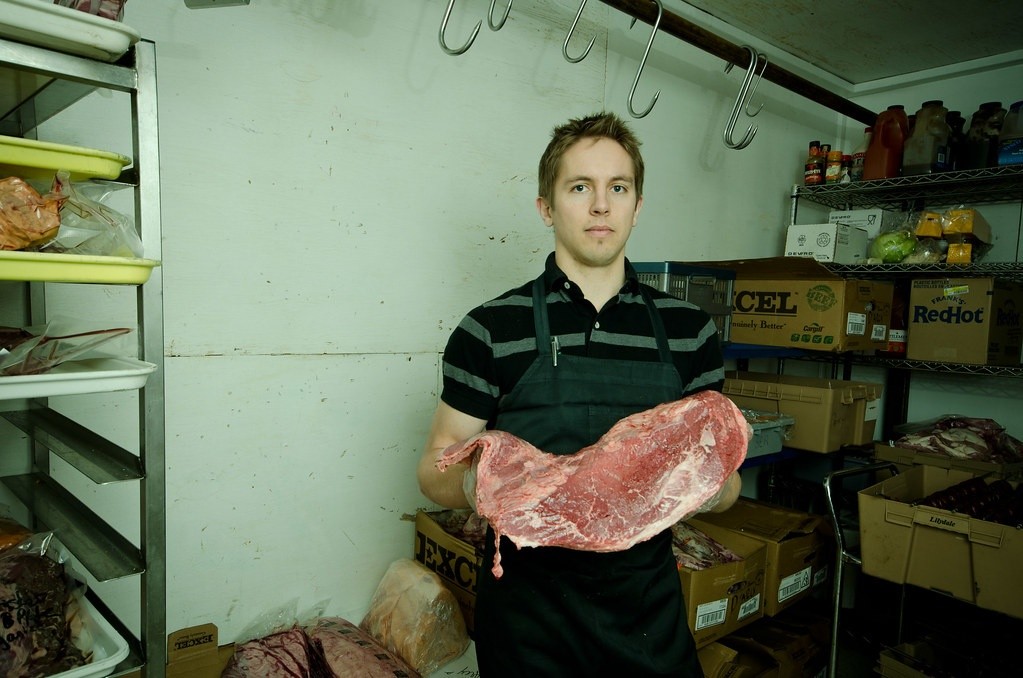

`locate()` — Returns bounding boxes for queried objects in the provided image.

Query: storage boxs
[784,222,868,265]
[721,370,885,452]
[413,510,484,642]
[629,262,734,343]
[729,278,893,353]
[906,277,995,365]
[828,209,895,244]
[692,495,828,618]
[676,518,769,649]
[858,463,1023,619]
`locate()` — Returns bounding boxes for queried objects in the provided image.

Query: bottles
[850,127,876,181]
[863,105,909,180]
[900,100,950,175]
[802,140,852,186]
[965,101,1006,171]
[996,101,1022,165]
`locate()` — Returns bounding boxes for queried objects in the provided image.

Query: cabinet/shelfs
[0,0,165,678]
[787,163,1023,678]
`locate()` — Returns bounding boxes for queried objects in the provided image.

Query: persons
[416,111,742,678]
